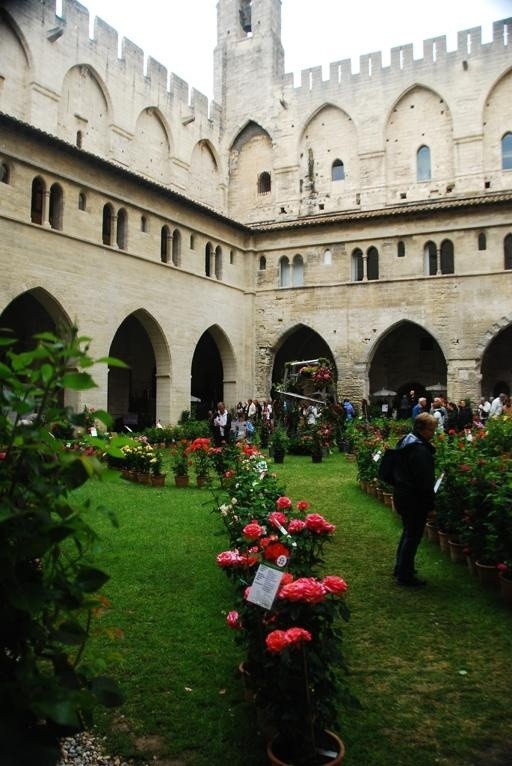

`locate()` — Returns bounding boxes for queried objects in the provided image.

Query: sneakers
[393,570,426,587]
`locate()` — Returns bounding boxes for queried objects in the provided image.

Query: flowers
[216,363,351,496]
[351,398,512,599]
[62,402,216,488]
[208,496,352,766]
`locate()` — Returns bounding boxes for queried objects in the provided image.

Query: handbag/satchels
[377,447,407,485]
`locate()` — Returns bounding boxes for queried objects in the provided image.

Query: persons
[361,398,369,422]
[394,412,439,584]
[207,399,323,470]
[335,402,344,417]
[343,399,355,423]
[369,387,512,435]
